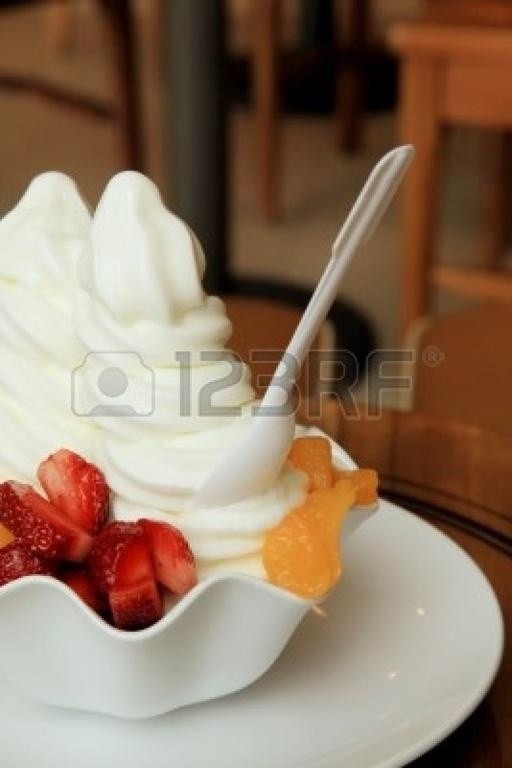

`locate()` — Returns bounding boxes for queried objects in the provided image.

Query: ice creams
[0,169,378,628]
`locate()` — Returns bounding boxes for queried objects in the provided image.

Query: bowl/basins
[0,419,382,722]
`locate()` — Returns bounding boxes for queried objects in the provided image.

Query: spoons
[189,144,421,510]
